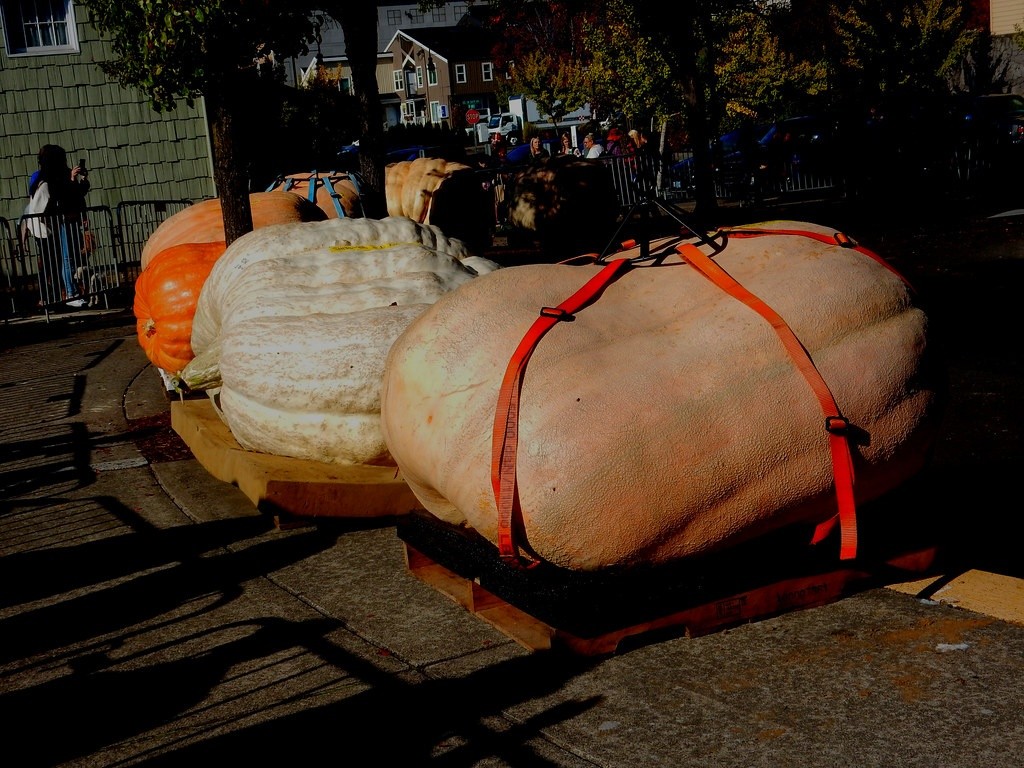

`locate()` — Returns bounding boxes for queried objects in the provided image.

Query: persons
[23,145,91,313]
[527,128,677,207]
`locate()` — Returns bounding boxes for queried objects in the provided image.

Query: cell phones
[79,158,85,175]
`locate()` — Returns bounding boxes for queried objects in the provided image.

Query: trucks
[490,93,598,142]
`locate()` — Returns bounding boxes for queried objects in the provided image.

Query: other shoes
[66,297,87,307]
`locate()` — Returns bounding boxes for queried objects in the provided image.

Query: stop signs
[466,110,481,124]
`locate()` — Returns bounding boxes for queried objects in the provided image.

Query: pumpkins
[134,152,940,577]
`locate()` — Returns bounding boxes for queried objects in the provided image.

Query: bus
[462,106,495,139]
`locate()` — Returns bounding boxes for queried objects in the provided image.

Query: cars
[671,104,821,196]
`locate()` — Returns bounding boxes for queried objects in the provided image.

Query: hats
[607,128,623,141]
